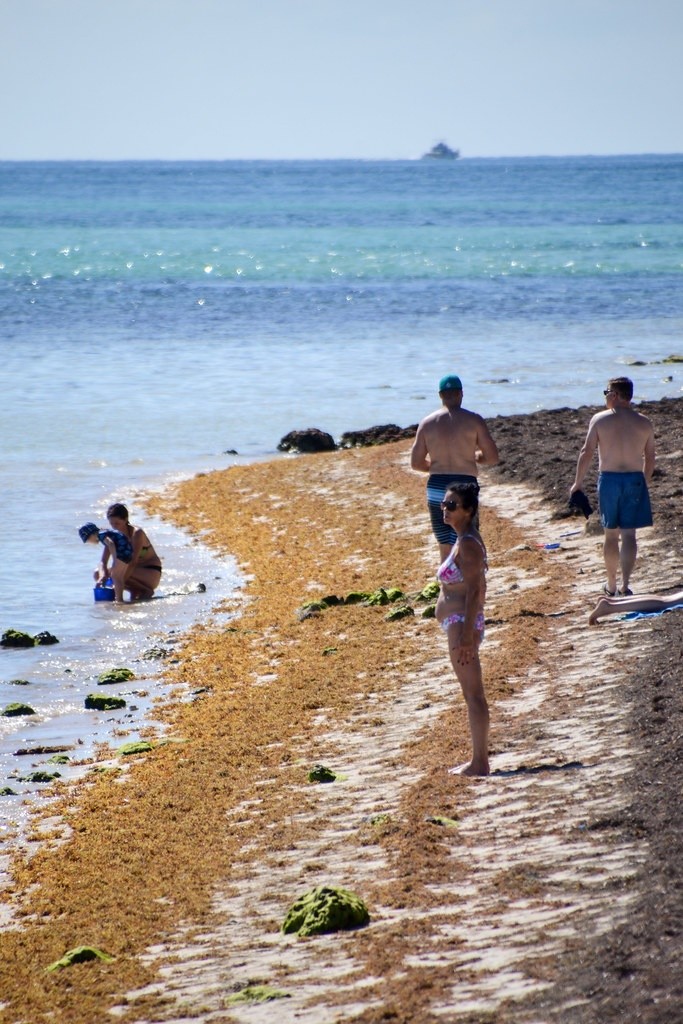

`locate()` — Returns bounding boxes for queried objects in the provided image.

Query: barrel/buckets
[94,588,115,600]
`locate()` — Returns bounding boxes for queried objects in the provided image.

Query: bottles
[546,543,559,548]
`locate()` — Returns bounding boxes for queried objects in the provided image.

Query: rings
[466,651,471,655]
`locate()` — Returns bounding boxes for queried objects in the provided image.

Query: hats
[78,522,100,543]
[438,375,462,392]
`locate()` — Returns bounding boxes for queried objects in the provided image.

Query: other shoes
[602,584,615,597]
[618,589,633,595]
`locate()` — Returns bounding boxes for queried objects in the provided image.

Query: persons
[411,375,499,566]
[431,484,490,776]
[570,377,656,597]
[79,503,161,603]
[588,591,683,626]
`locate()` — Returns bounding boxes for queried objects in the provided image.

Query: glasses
[604,390,619,395]
[440,501,463,512]
